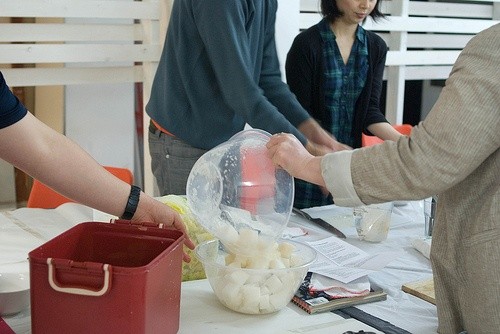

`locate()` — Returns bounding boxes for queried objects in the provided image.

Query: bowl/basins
[195,238,317,316]
[186,129,296,259]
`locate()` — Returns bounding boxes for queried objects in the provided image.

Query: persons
[275,0,403,213]
[266,21,500,334]
[0,71,196,262]
[144,0,354,249]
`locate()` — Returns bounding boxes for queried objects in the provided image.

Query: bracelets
[120,184,142,220]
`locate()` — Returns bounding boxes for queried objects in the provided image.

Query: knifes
[292,206,346,240]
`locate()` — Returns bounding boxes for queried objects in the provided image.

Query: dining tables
[0,196,438,334]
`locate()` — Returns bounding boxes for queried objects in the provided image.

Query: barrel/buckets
[29,217,185,333]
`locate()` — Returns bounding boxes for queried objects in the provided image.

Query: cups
[353,200,393,243]
[422,196,437,237]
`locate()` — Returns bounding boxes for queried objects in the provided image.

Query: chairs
[26,167,133,207]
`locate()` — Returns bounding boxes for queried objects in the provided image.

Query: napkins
[307,274,397,297]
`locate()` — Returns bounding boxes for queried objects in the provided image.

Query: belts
[149,120,163,138]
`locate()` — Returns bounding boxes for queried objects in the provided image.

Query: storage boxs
[29,219,185,334]
